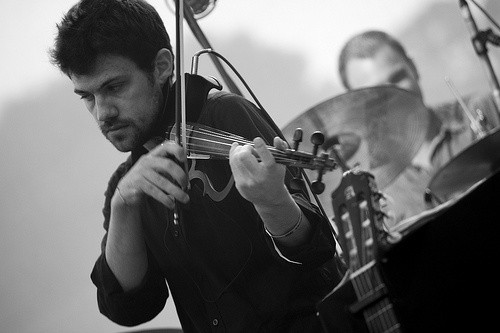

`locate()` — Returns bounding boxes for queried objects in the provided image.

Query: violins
[128,74,336,202]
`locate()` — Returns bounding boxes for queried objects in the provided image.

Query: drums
[317,171,499,332]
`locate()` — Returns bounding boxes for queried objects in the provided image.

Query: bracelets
[114,181,138,207]
[264,207,303,240]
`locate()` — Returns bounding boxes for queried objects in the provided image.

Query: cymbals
[428,125,500,198]
[280,85,431,213]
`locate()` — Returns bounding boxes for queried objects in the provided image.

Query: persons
[48,0,337,333]
[337,31,500,229]
